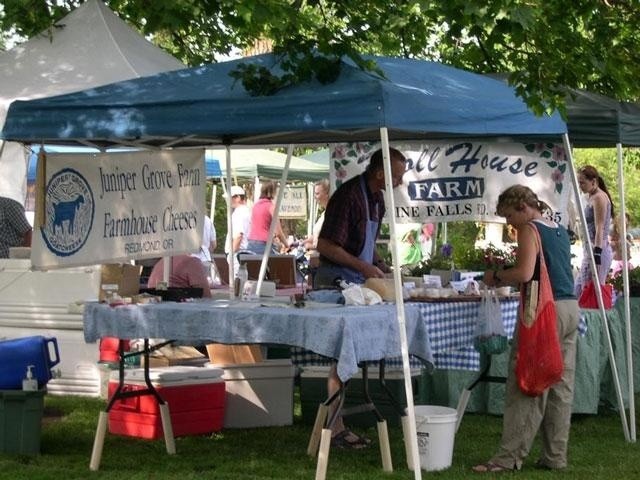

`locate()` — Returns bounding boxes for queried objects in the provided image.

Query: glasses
[577,167,586,173]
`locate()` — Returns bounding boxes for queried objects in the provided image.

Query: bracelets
[374,259,385,266]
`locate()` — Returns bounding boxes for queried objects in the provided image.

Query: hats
[222,186,244,198]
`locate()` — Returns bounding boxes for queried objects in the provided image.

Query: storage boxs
[107,367,225,440]
[298,363,420,426]
[0,387,47,461]
[204,361,295,429]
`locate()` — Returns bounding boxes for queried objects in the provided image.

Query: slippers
[471,461,512,473]
[533,458,550,470]
[331,427,370,449]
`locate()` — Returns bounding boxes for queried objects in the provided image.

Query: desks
[290,295,587,437]
[562,307,629,415]
[292,294,521,457]
[79,298,435,480]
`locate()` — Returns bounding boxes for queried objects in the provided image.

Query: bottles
[234,262,248,298]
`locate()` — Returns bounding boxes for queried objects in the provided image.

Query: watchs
[493,270,501,282]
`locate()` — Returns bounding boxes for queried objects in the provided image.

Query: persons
[312,146,406,449]
[0,195,32,259]
[418,223,434,269]
[401,228,423,270]
[468,184,579,472]
[573,166,634,306]
[140,178,330,359]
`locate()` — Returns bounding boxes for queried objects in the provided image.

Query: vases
[431,268,452,285]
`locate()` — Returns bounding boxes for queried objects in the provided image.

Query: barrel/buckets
[0,389,47,457]
[401,405,457,471]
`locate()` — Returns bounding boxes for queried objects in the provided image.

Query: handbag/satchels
[578,282,613,309]
[514,301,564,396]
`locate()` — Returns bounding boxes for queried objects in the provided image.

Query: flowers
[429,242,456,271]
